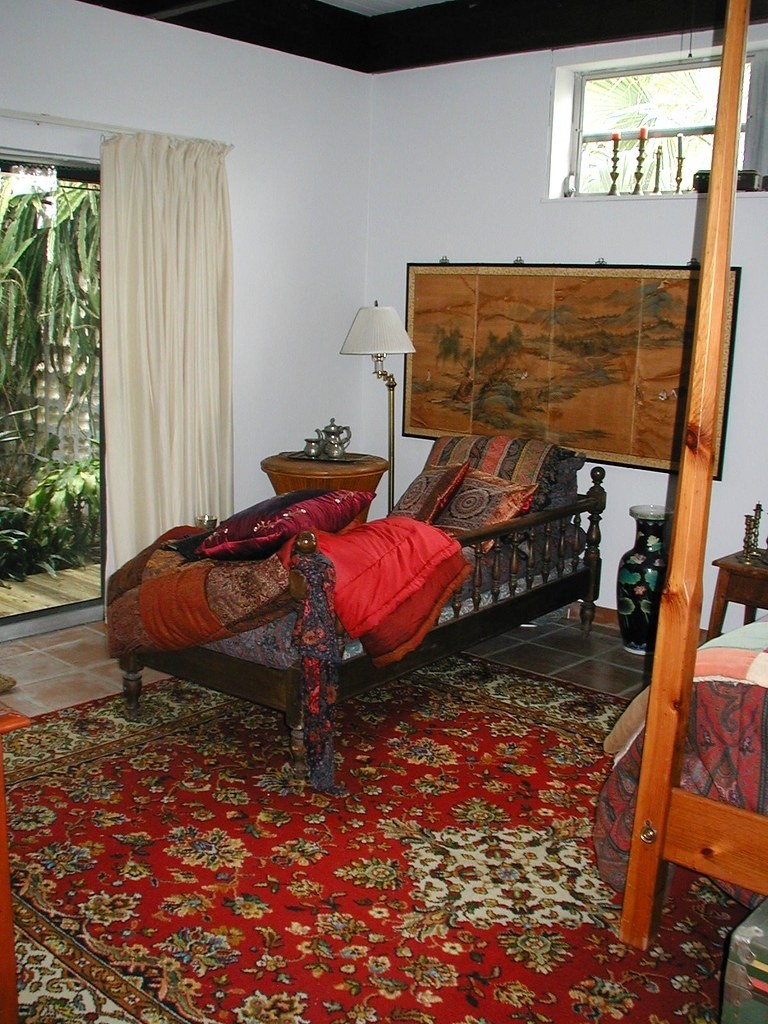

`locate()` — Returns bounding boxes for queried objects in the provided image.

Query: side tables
[259,448,389,524]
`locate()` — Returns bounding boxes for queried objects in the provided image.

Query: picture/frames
[401,262,743,482]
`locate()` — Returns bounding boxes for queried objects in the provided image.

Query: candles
[639,128,649,140]
[657,144,663,153]
[676,133,684,157]
[612,132,622,138]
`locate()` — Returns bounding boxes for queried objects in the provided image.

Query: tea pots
[315,417,352,453]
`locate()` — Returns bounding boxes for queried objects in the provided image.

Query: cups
[304,438,321,458]
[327,440,345,458]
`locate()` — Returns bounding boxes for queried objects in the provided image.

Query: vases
[617,505,668,656]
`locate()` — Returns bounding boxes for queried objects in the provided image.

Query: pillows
[383,459,471,525]
[161,489,376,561]
[422,434,577,550]
[434,466,541,556]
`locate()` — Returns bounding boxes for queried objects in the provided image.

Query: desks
[706,547,768,643]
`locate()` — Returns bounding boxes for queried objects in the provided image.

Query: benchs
[103,425,606,789]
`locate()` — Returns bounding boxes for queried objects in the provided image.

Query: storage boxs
[719,896,768,1024]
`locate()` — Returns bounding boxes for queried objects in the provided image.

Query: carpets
[0,648,751,1024]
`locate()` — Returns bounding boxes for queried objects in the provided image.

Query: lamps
[339,301,417,511]
